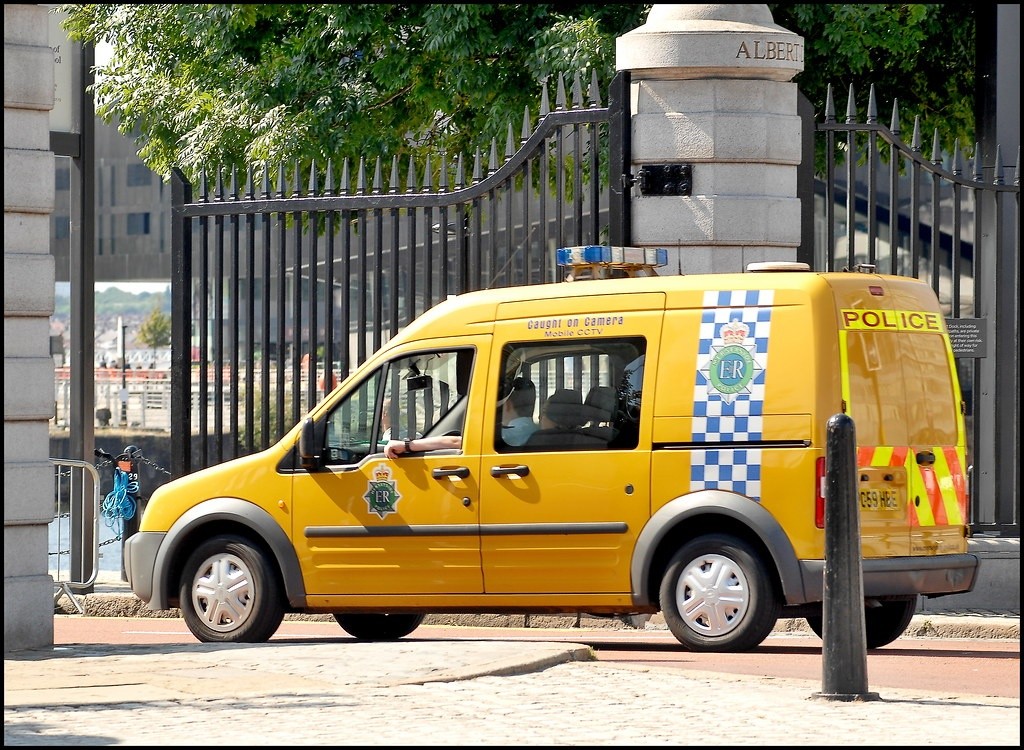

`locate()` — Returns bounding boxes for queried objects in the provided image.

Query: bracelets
[404,438,412,453]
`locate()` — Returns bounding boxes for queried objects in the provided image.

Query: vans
[121,244,981,648]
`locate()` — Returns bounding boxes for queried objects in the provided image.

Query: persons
[382,398,406,440]
[383,377,539,460]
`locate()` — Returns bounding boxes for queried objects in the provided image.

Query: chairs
[528,384,617,448]
